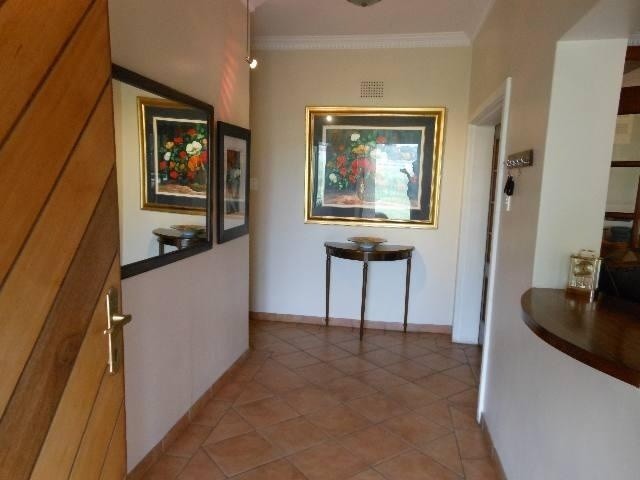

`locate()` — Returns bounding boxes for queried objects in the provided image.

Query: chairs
[601,239,640,297]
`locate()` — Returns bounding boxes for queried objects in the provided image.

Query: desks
[324,243,415,341]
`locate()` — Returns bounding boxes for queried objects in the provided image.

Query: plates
[348,236,387,248]
[170,224,205,236]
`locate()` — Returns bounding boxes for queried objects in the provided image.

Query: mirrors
[111,62,214,280]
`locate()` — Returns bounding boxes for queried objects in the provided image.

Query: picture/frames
[304,106,446,230]
[136,96,207,216]
[217,121,250,244]
